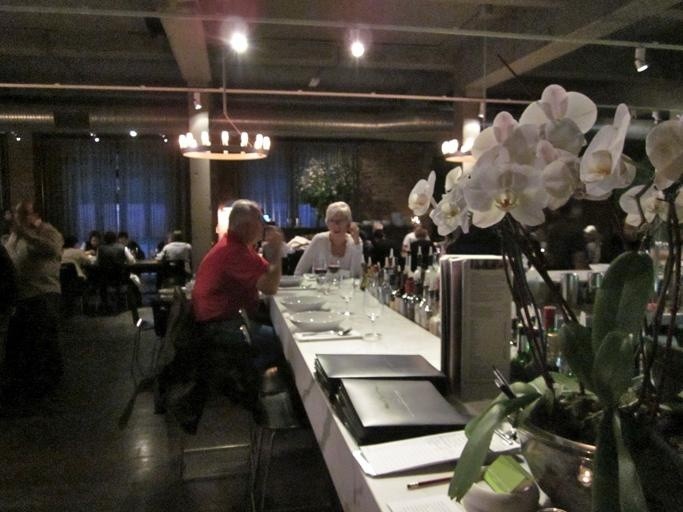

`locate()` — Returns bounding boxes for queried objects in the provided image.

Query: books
[332,379,477,446]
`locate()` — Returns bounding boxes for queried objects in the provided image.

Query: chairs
[121,273,312,511]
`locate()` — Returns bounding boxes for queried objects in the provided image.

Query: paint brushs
[492,365,525,410]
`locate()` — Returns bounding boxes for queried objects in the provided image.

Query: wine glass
[314,251,380,342]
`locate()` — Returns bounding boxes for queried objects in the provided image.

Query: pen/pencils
[407,477,453,488]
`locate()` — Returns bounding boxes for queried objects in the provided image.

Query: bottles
[364,260,444,331]
[512,301,577,377]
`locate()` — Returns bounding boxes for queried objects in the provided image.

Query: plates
[276,272,341,328]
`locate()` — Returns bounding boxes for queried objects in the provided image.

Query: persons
[446,224,503,269]
[360,231,401,266]
[401,221,436,271]
[1,199,64,402]
[294,201,365,279]
[154,230,193,293]
[60,231,145,317]
[528,221,576,269]
[438,233,455,257]
[574,224,613,270]
[192,199,301,423]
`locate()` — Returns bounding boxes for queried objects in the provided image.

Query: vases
[510,395,598,509]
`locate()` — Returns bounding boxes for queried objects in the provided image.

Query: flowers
[405,84,683,511]
